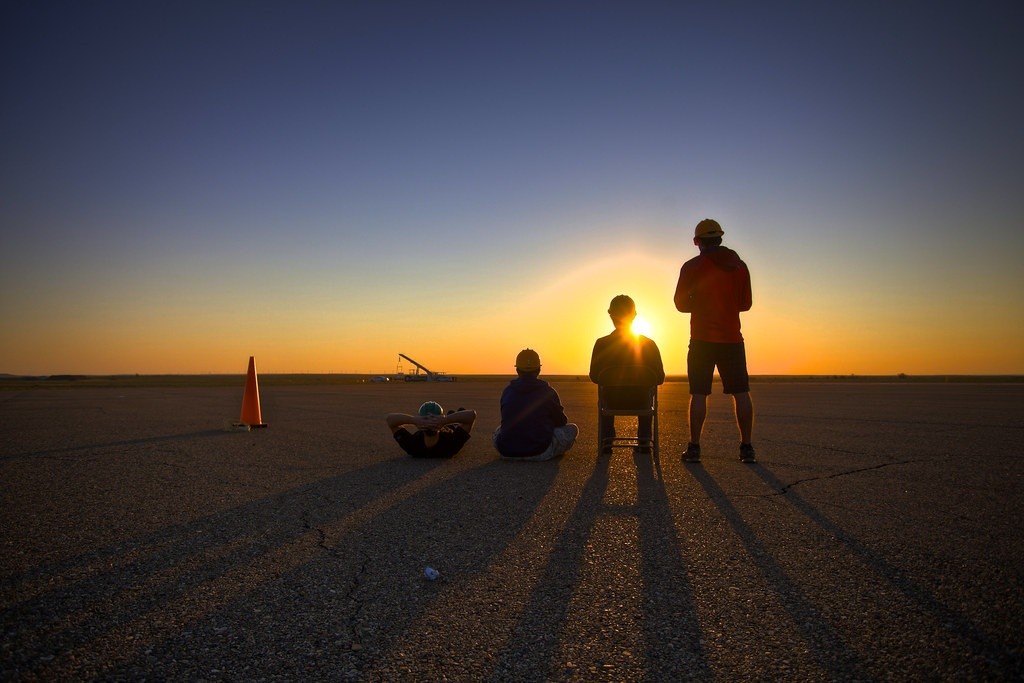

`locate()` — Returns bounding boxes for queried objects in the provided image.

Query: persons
[674,219,756,462]
[493,348,579,461]
[589,294,665,453]
[386,401,477,457]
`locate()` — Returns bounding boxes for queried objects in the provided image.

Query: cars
[370,376,390,382]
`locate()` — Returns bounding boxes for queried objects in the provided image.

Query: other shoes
[739,443,755,463]
[682,443,702,462]
[634,443,650,453]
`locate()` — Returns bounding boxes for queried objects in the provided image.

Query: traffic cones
[232,354,268,429]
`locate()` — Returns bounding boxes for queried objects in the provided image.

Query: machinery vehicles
[393,354,458,382]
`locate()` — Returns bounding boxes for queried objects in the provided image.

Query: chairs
[597,363,660,469]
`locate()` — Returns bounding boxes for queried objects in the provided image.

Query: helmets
[418,402,443,416]
[695,219,724,237]
[514,348,542,371]
[608,294,637,322]
